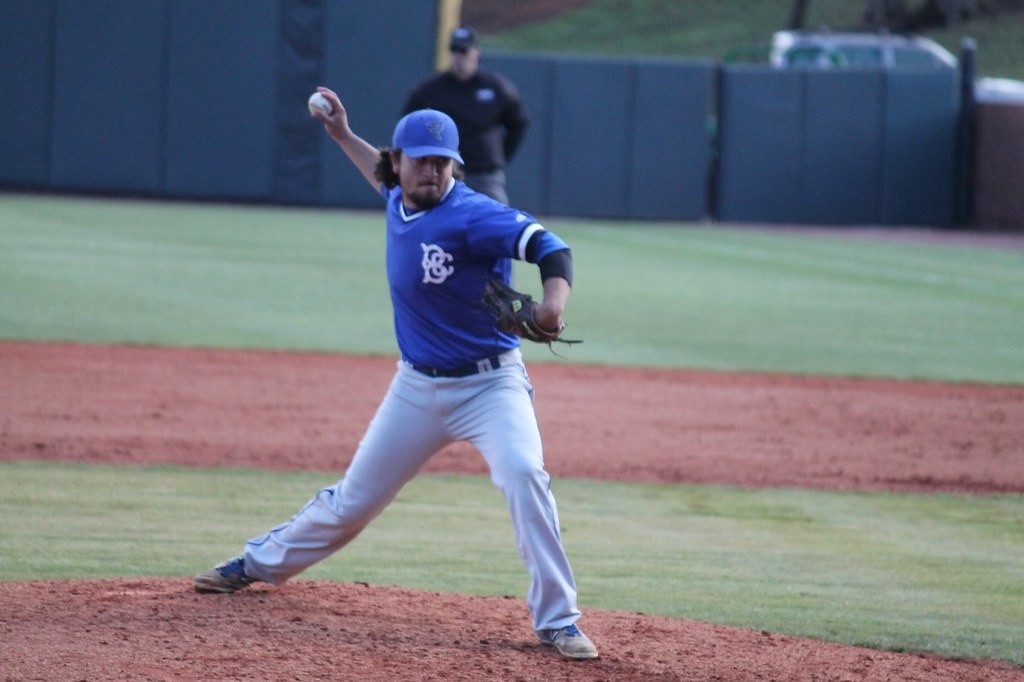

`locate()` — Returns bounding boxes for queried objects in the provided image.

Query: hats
[393,108,465,165]
[450,26,478,48]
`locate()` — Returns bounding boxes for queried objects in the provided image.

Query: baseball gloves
[479,277,569,345]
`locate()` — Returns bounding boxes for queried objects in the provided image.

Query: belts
[401,354,500,377]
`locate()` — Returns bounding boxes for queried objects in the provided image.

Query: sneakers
[536,622,598,659]
[194,554,256,593]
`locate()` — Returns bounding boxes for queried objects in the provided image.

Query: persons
[194,86,599,658]
[401,27,531,207]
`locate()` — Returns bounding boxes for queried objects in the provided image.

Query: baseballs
[307,90,335,117]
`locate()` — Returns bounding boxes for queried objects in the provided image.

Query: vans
[770,30,960,78]
